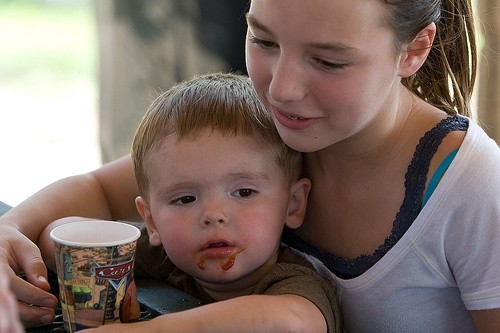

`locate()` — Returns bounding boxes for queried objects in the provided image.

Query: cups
[49,219,142,333]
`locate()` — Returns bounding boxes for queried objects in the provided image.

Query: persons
[2,1,498,333]
[36,72,342,333]
